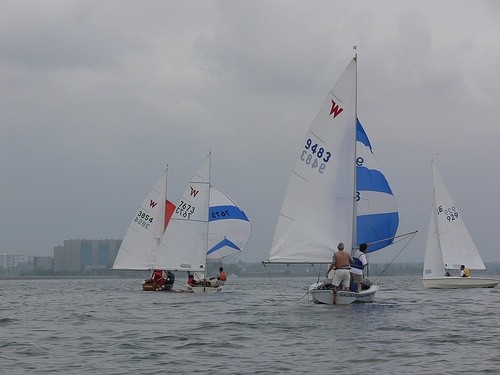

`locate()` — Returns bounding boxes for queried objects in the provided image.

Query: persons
[349,243,368,292]
[326,243,355,291]
[459,264,470,277]
[216,267,227,286]
[188,275,196,286]
[151,269,175,290]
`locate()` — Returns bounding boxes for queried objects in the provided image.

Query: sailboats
[260,45,419,306]
[108,150,254,297]
[421,152,500,290]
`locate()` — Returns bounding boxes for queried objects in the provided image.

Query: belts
[333,268,351,270]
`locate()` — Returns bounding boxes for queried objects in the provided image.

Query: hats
[337,243,344,249]
